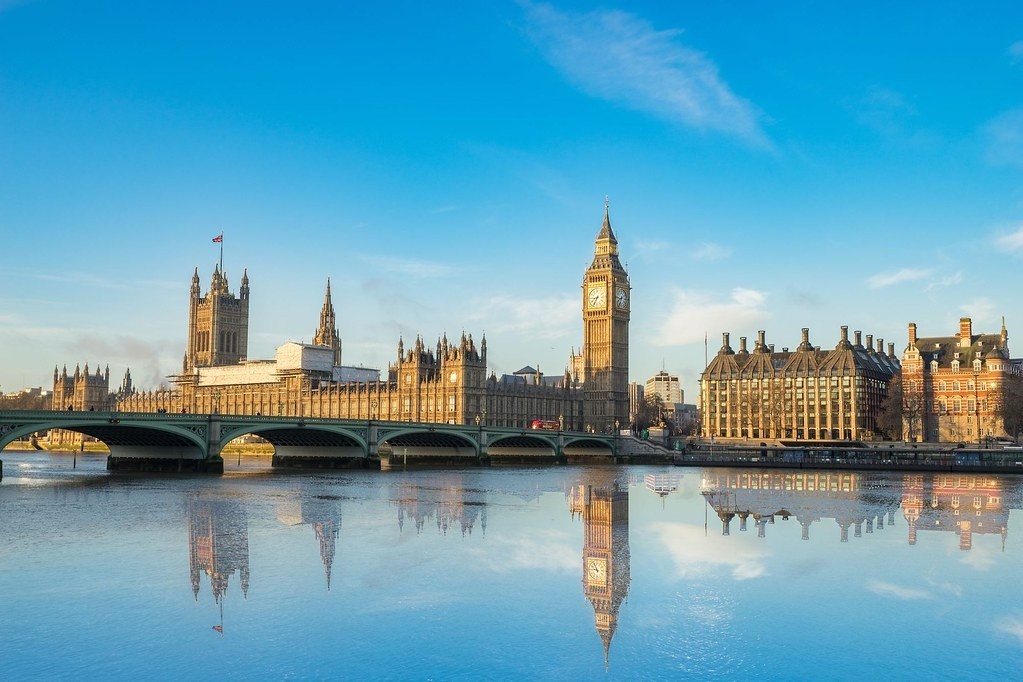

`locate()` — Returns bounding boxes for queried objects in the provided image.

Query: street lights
[475,415,480,425]
[393,408,399,421]
[371,400,378,419]
[559,414,564,431]
[278,401,284,416]
[115,394,124,412]
[587,425,590,433]
[213,387,221,414]
[615,419,619,433]
[481,409,486,426]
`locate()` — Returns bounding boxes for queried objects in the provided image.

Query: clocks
[589,288,605,307]
[589,562,604,580]
[617,288,627,308]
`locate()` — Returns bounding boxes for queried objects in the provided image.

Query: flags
[213,235,222,242]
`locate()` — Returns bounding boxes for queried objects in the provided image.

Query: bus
[532,420,559,430]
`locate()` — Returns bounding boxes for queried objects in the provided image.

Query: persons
[257,412,260,415]
[68,405,73,410]
[182,408,186,413]
[90,406,94,410]
[158,409,166,413]
